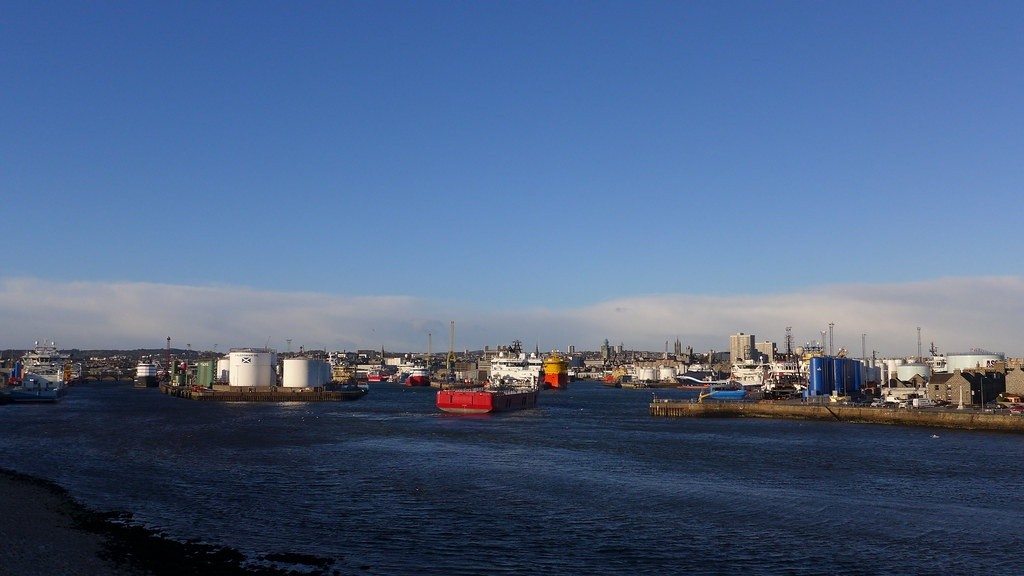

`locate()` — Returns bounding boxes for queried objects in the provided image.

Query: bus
[1004,396,1020,405]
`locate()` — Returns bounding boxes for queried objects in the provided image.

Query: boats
[366,370,383,382]
[701,384,748,401]
[405,368,431,387]
[158,380,369,402]
[436,387,539,415]
[0,339,87,403]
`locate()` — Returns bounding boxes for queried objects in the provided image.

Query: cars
[1007,405,1023,416]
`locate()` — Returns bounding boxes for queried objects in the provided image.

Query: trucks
[912,397,931,409]
